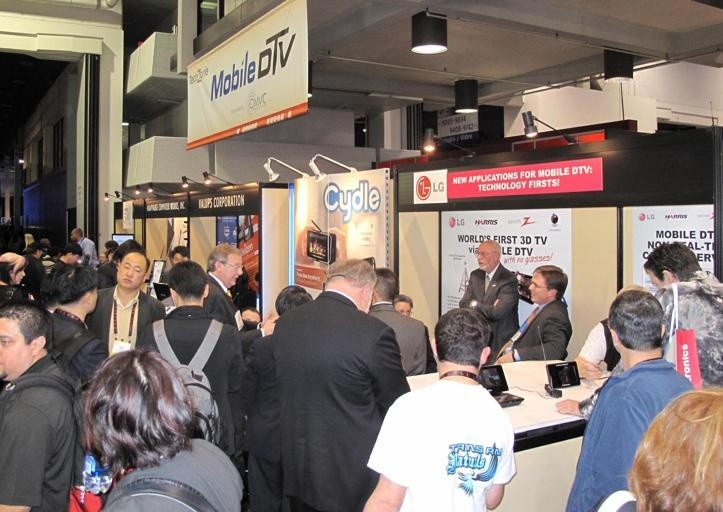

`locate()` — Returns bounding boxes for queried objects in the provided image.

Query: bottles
[82,452,113,494]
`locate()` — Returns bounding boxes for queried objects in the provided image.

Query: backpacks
[173,364,225,448]
[42,337,92,390]
[67,393,116,512]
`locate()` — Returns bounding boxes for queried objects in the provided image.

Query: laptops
[478,364,524,407]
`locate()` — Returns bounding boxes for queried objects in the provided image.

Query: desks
[406,358,615,511]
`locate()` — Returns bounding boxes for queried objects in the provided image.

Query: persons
[453,238,573,364]
[563,241,722,512]
[1,228,520,512]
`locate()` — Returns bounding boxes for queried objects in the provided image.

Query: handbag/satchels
[661,327,704,391]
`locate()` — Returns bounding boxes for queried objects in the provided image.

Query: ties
[494,307,546,361]
[484,274,490,294]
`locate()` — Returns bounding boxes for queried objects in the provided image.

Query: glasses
[474,250,496,258]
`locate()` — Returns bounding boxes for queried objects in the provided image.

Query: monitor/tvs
[112,233,134,247]
[153,282,171,300]
[306,229,336,265]
[546,361,580,389]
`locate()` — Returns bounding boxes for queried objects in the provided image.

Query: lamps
[308,60,312,98]
[604,49,633,83]
[411,12,447,55]
[182,176,206,188]
[422,128,475,157]
[262,156,308,183]
[453,79,478,114]
[309,154,356,180]
[203,171,234,186]
[104,182,174,201]
[522,111,576,145]
